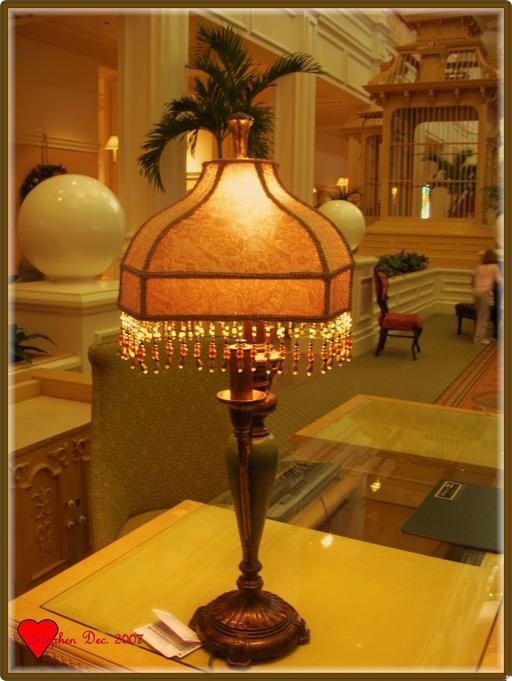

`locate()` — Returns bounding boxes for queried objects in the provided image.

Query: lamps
[116,111,352,664]
[104,133,121,163]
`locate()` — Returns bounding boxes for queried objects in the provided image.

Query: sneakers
[475,339,490,345]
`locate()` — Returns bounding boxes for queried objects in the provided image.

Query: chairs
[373,270,423,360]
[454,282,497,338]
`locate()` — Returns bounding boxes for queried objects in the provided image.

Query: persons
[469,248,503,343]
